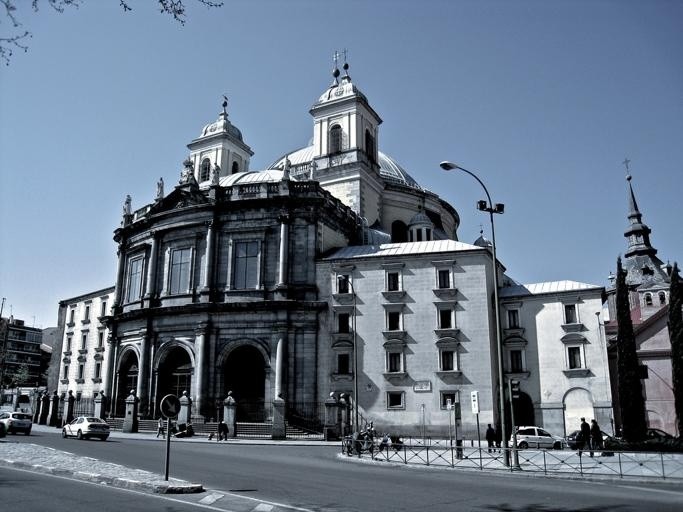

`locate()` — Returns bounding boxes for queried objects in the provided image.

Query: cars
[62,414,110,441]
[0,410,32,438]
[507,425,682,452]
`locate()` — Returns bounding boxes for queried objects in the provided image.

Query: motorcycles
[344,428,402,453]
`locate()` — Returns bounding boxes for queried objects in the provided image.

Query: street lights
[336,274,358,433]
[439,161,508,468]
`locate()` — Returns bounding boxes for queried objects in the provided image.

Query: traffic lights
[510,379,520,401]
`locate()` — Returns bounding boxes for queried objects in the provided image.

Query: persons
[218,419,222,441]
[221,421,228,440]
[589,419,602,450]
[575,417,593,456]
[207,431,214,440]
[494,424,501,452]
[485,423,495,452]
[155,416,166,440]
